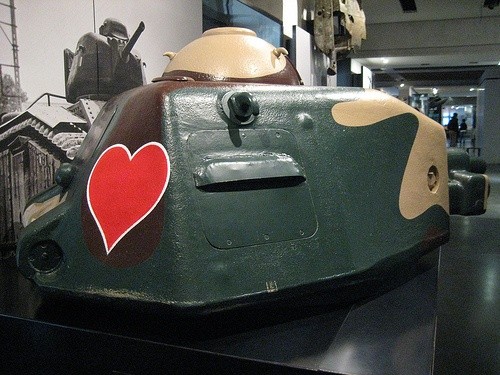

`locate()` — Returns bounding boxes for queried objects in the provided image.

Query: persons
[447,113,460,147]
[458,118,468,149]
